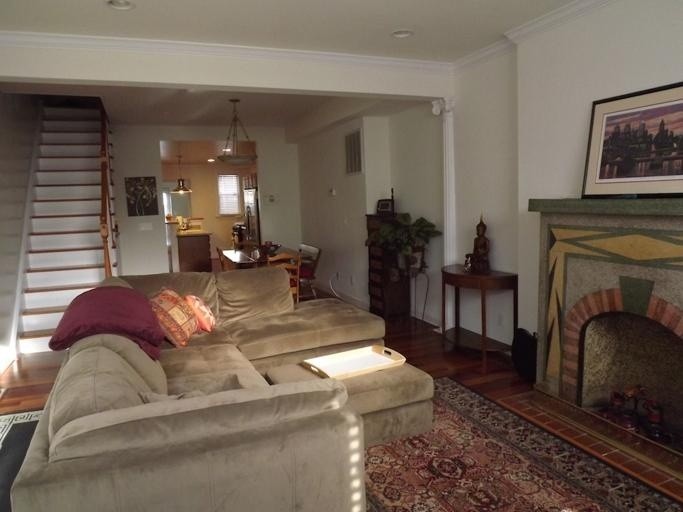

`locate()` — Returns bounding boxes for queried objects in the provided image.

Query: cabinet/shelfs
[364,213,410,321]
[175,232,212,273]
[243,188,260,249]
[440,264,518,374]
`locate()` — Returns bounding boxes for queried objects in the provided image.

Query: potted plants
[363,212,443,272]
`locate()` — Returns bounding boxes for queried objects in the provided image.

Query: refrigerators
[242,186,259,245]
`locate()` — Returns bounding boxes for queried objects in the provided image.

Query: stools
[265,361,434,450]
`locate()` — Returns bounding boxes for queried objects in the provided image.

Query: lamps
[216,99,258,165]
[169,155,192,195]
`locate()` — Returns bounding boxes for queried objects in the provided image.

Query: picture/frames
[580,81,683,198]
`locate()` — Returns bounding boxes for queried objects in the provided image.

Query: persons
[469,222,490,276]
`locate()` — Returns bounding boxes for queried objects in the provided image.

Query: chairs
[215,243,321,303]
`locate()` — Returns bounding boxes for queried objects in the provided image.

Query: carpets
[362,377,682,512]
